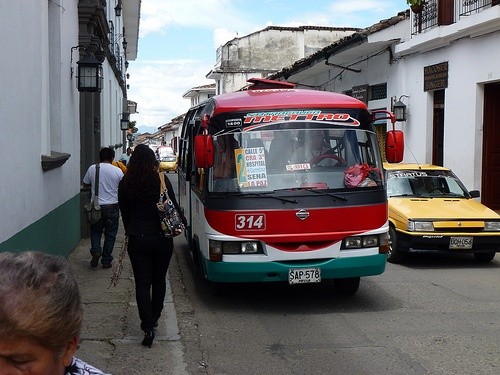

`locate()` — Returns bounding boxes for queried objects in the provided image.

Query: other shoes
[90,252,100,267]
[103,262,112,268]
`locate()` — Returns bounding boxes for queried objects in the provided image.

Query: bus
[177,77,405,299]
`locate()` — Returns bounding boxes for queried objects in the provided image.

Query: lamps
[126,73,130,89]
[114,4,123,17]
[127,133,132,140]
[117,113,129,130]
[70,44,102,92]
[125,60,129,68]
[122,38,127,49]
[392,95,409,122]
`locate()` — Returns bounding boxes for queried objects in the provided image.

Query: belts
[100,204,117,206]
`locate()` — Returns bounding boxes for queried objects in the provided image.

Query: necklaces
[310,150,322,165]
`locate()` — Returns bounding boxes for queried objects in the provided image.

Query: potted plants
[406,0,423,14]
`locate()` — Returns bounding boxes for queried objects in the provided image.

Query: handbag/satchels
[83,196,101,224]
[156,172,185,238]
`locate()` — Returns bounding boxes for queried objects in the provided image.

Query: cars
[382,162,500,265]
[148,144,178,174]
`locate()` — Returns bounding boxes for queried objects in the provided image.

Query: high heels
[152,322,158,329]
[142,331,154,348]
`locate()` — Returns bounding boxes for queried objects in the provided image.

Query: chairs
[247,130,337,173]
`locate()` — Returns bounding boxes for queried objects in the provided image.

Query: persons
[118,144,186,348]
[84,148,124,268]
[288,130,343,168]
[120,148,132,166]
[0,251,110,375]
[213,134,233,181]
